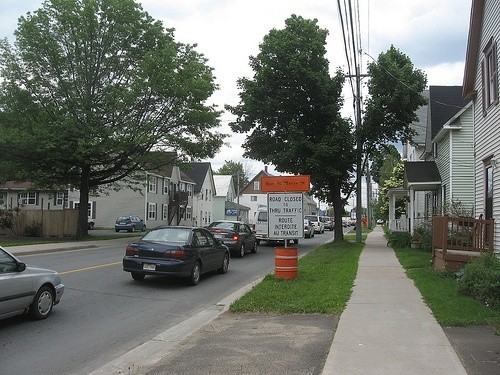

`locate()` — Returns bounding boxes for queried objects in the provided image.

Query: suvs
[320,217,335,231]
[304,214,325,234]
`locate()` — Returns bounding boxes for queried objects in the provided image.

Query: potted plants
[410,232,422,249]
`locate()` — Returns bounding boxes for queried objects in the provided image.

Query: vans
[256,206,300,245]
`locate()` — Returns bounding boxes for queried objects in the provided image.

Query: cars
[122,225,231,286]
[303,220,317,236]
[0,246,65,323]
[114,215,147,232]
[206,221,258,257]
[341,217,356,227]
[88,216,95,230]
